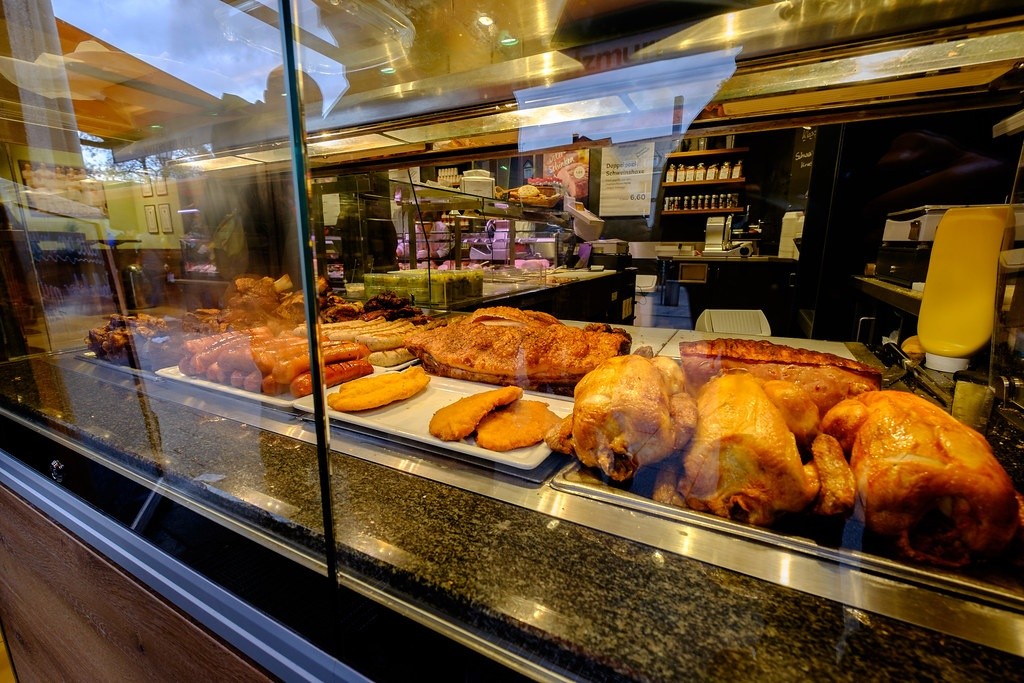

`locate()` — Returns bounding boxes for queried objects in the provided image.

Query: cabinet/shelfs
[659,135,746,214]
[399,148,602,269]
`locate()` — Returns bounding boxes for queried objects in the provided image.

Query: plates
[85,242,865,472]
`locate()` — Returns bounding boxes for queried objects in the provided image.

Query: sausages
[176,313,449,398]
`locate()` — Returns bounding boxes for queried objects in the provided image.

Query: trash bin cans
[634,274,659,327]
[122,263,158,309]
[694,310,771,336]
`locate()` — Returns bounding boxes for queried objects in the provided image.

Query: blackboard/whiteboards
[0,175,164,454]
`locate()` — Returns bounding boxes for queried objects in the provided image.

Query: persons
[212,67,324,292]
[731,170,782,255]
[21,162,93,187]
[0,202,41,410]
[395,208,456,262]
[515,218,535,258]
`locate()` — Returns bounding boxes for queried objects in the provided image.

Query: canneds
[664,192,737,210]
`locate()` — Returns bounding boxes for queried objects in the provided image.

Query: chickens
[650,368,820,527]
[810,388,1024,566]
[543,355,697,480]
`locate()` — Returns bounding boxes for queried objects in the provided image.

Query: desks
[574,251,796,337]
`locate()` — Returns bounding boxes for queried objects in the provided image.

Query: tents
[0,177,168,479]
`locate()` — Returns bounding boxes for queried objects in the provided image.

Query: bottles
[665,161,744,183]
